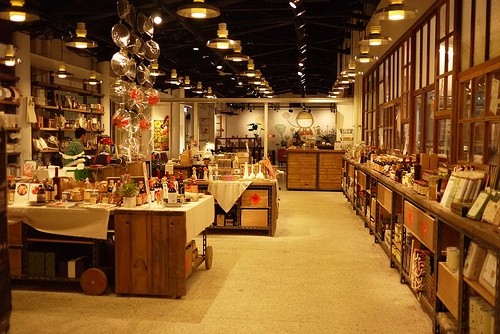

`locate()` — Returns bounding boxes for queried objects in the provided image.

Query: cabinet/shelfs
[285,145,346,192]
[342,155,500,334]
[0,30,278,298]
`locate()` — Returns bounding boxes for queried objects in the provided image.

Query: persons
[63,128,87,180]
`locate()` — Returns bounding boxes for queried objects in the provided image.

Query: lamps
[0,0,419,99]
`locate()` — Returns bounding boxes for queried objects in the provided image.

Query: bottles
[360,146,415,182]
[36,166,198,208]
